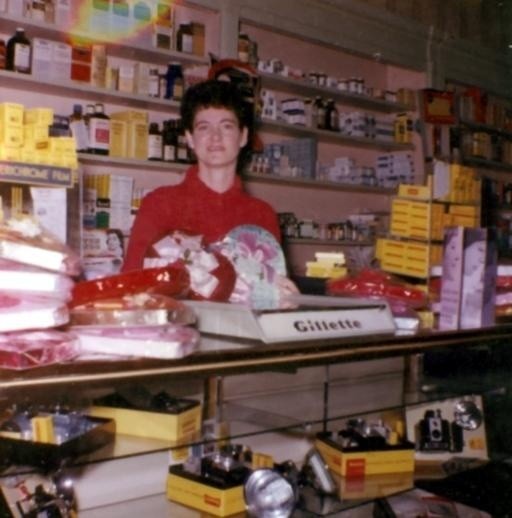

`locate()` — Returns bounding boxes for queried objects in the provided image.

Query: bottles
[0,24,32,73]
[69,104,109,155]
[325,222,359,241]
[423,155,433,184]
[148,118,192,163]
[363,112,376,138]
[394,113,411,143]
[148,61,185,101]
[239,32,364,96]
[246,155,270,175]
[176,19,193,54]
[303,96,340,131]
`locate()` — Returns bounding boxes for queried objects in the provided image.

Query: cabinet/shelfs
[0,0,418,246]
[2,325,512,474]
[449,117,510,261]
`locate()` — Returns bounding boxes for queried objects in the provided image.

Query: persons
[102,229,125,256]
[121,79,283,272]
